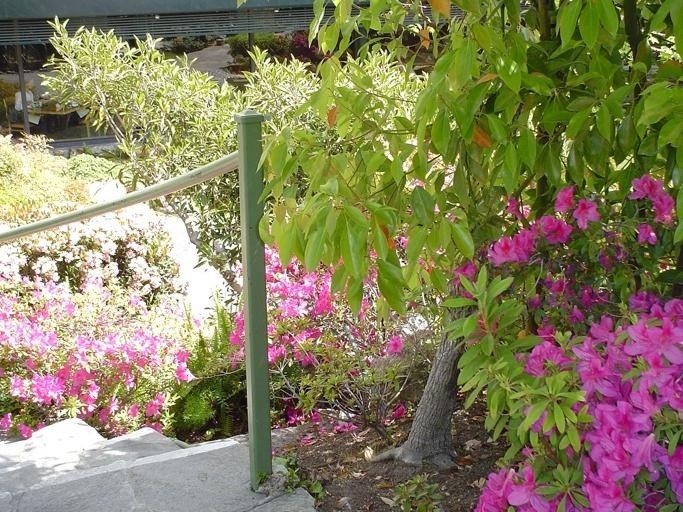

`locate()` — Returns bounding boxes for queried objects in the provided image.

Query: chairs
[3,99,34,137]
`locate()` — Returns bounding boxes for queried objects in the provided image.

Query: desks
[26,100,77,131]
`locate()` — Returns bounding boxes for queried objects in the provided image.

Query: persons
[14,79,48,134]
[68,108,90,137]
